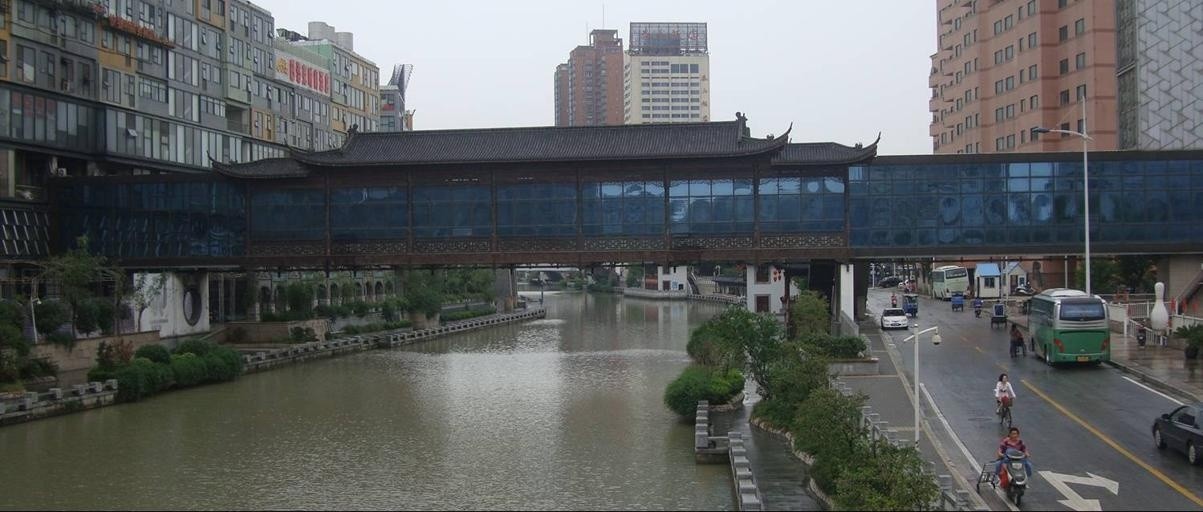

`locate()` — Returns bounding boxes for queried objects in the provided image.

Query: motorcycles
[951,290,963,311]
[891,294,898,307]
[1016,280,1038,296]
[974,299,984,318]
[901,293,918,317]
[902,287,913,294]
[1136,318,1149,347]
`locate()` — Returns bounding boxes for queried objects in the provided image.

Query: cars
[879,276,902,287]
[879,308,908,330]
[994,447,1030,505]
[898,279,916,287]
[1151,402,1202,466]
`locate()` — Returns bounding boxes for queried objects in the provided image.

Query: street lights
[30,295,42,342]
[1029,95,1093,296]
[903,321,943,449]
[539,271,544,302]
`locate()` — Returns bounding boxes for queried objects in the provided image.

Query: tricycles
[991,303,1009,331]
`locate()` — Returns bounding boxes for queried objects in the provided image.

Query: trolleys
[977,460,999,498]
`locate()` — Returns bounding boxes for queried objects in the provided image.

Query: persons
[996,428,1032,485]
[974,297,983,308]
[890,293,896,300]
[1138,323,1145,333]
[903,281,915,292]
[1010,325,1016,358]
[995,373,1015,413]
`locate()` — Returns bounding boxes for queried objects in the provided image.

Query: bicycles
[995,395,1015,430]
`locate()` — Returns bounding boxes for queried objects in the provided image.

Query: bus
[1022,287,1111,367]
[926,265,971,299]
